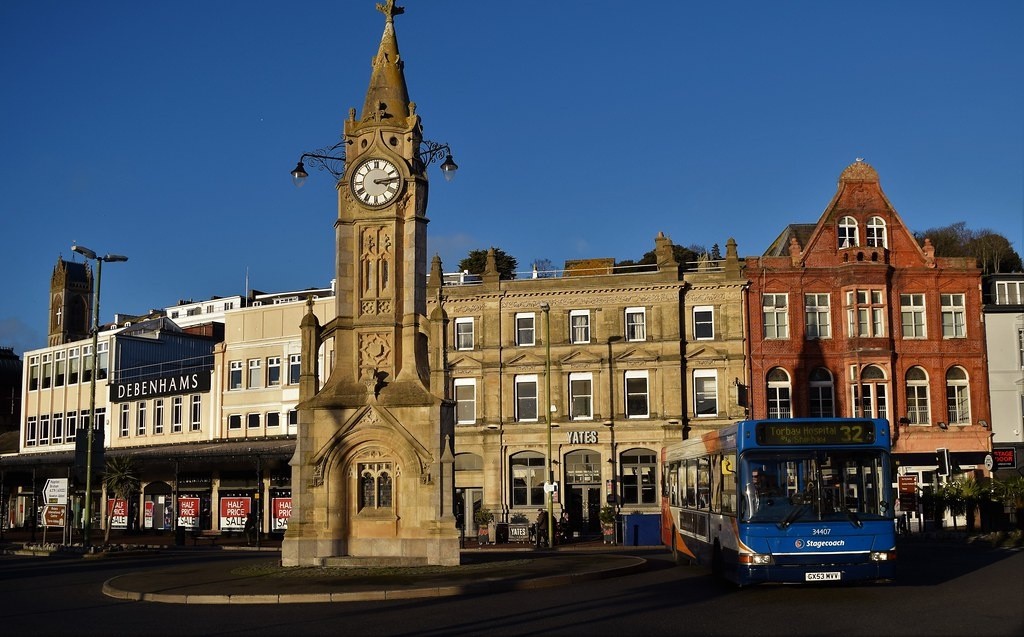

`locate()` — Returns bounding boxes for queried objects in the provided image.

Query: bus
[659,417,896,590]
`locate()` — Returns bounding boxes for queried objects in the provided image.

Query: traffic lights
[932,449,949,476]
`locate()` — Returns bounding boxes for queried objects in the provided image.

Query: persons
[752,470,782,496]
[536,508,548,547]
[245,513,255,545]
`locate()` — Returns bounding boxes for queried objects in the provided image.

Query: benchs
[192,530,222,545]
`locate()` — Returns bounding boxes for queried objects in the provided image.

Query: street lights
[70,244,129,547]
[539,302,555,545]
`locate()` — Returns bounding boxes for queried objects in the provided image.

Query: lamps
[291,153,347,188]
[420,142,458,184]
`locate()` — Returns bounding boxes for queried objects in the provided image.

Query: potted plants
[599,507,615,545]
[475,508,494,545]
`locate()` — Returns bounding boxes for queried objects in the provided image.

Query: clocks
[350,157,400,207]
